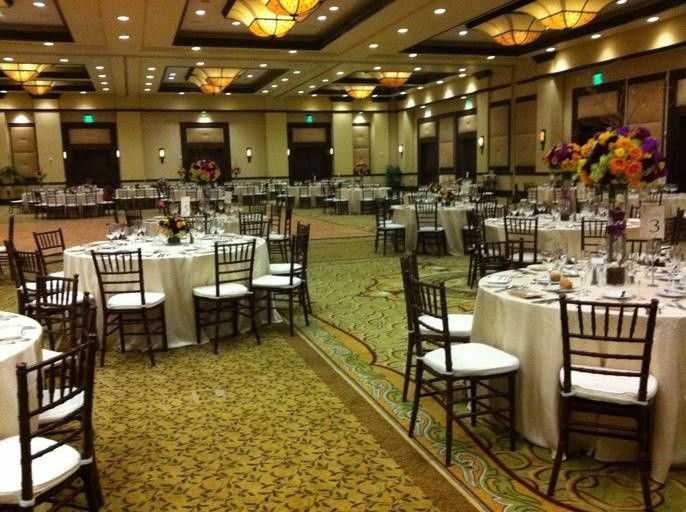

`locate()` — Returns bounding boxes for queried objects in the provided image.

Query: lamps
[473,11,538,47]
[346,86,378,102]
[220,0,320,40]
[186,68,246,94]
[0,63,57,97]
[379,71,412,91]
[518,0,616,34]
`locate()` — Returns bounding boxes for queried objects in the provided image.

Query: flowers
[188,159,222,186]
[353,162,371,177]
[545,143,584,185]
[578,126,670,190]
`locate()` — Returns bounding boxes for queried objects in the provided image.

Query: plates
[488,250,685,311]
[80,217,252,256]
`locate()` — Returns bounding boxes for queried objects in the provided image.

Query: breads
[550,269,561,281]
[560,278,572,289]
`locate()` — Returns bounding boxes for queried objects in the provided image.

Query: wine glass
[541,238,570,279]
[645,237,663,287]
